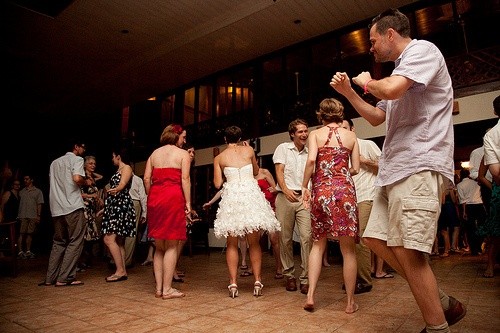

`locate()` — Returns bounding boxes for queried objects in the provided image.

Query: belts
[278,190,303,196]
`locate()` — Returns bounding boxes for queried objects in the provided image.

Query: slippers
[240,270,252,277]
[105,273,128,281]
[240,264,248,269]
[44,279,83,286]
[371,271,394,278]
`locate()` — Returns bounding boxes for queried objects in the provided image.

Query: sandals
[482,265,494,277]
[274,271,284,279]
[176,271,185,277]
[154,287,185,299]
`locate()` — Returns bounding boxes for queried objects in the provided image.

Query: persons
[0,175,44,258]
[339,119,395,295]
[273,118,314,294]
[144,124,199,300]
[44,142,154,287]
[329,9,467,333]
[430,169,488,258]
[469,95,500,278]
[302,98,359,314]
[202,125,285,298]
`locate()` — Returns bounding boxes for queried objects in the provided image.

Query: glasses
[88,163,96,165]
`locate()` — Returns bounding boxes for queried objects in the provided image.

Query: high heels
[253,281,264,298]
[227,284,239,299]
[141,256,153,266]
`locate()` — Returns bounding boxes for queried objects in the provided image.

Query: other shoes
[173,275,184,283]
[286,277,297,291]
[428,246,471,257]
[343,279,372,294]
[420,296,466,333]
[17,250,32,258]
[300,284,309,294]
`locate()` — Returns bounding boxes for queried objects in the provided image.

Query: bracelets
[364,79,373,94]
